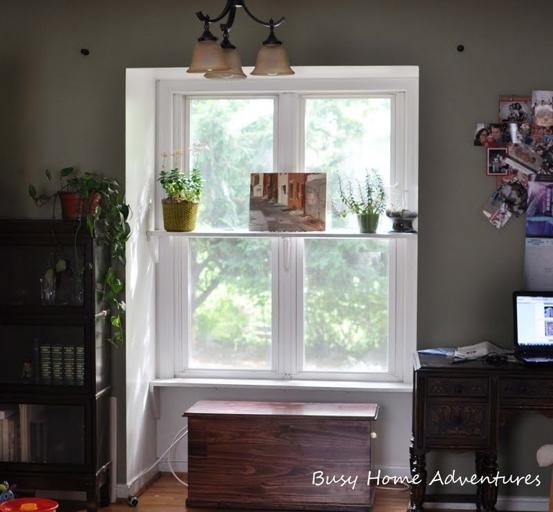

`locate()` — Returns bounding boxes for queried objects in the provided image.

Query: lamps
[188,0,296,78]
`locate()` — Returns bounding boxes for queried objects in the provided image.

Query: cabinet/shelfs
[1,214,114,507]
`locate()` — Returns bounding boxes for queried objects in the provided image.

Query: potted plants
[28,165,132,351]
[157,164,205,232]
[335,167,399,233]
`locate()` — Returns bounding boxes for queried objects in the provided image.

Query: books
[0,402,49,464]
[29,334,86,385]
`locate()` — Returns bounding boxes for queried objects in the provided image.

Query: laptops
[512,291,553,365]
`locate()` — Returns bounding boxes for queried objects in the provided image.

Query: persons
[473,89,552,229]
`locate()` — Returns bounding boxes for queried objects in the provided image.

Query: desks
[409,364,552,511]
